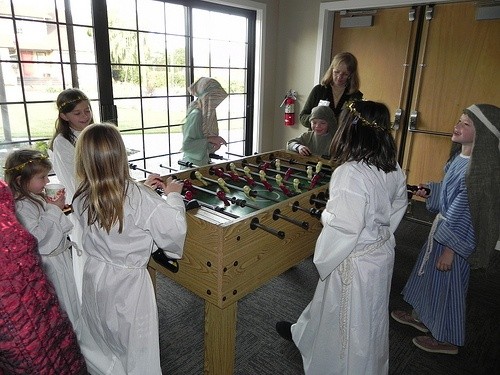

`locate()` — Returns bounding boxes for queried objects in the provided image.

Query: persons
[298,51,365,131]
[0,178,88,375]
[4,148,76,328]
[179,76,228,170]
[284,105,339,158]
[392,105,500,354]
[274,98,409,375]
[70,122,189,375]
[50,88,94,209]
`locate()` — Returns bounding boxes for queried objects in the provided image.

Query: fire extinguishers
[279,90,297,127]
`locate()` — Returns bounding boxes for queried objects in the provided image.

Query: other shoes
[276,320,296,343]
[412,335,459,355]
[390,309,429,332]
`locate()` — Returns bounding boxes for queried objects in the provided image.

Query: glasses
[331,70,353,77]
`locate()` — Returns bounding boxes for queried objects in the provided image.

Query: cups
[44,184,65,202]
